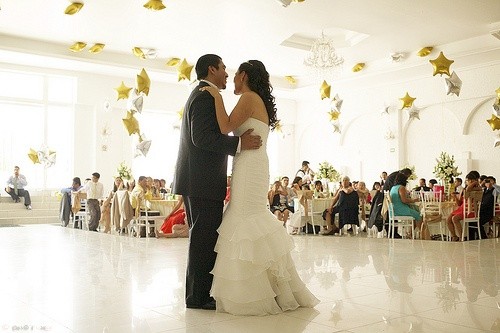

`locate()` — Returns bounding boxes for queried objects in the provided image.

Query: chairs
[60,189,500,242]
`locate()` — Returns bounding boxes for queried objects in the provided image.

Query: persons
[170,54,263,310]
[199,60,322,316]
[61,173,232,238]
[266,161,500,242]
[4,166,33,210]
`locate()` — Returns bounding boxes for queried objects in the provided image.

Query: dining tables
[145,200,185,217]
[292,198,337,227]
[397,202,469,238]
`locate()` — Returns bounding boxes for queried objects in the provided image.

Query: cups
[315,192,318,197]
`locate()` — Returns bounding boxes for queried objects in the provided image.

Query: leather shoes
[186,300,216,309]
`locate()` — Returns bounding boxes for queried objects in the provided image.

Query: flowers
[404,166,418,182]
[314,161,341,183]
[431,153,462,185]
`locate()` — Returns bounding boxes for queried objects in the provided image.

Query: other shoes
[452,236,459,241]
[334,228,339,233]
[16,198,21,202]
[387,233,401,238]
[322,229,333,236]
[26,205,32,209]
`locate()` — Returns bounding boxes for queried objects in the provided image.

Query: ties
[93,183,97,197]
[14,177,19,195]
